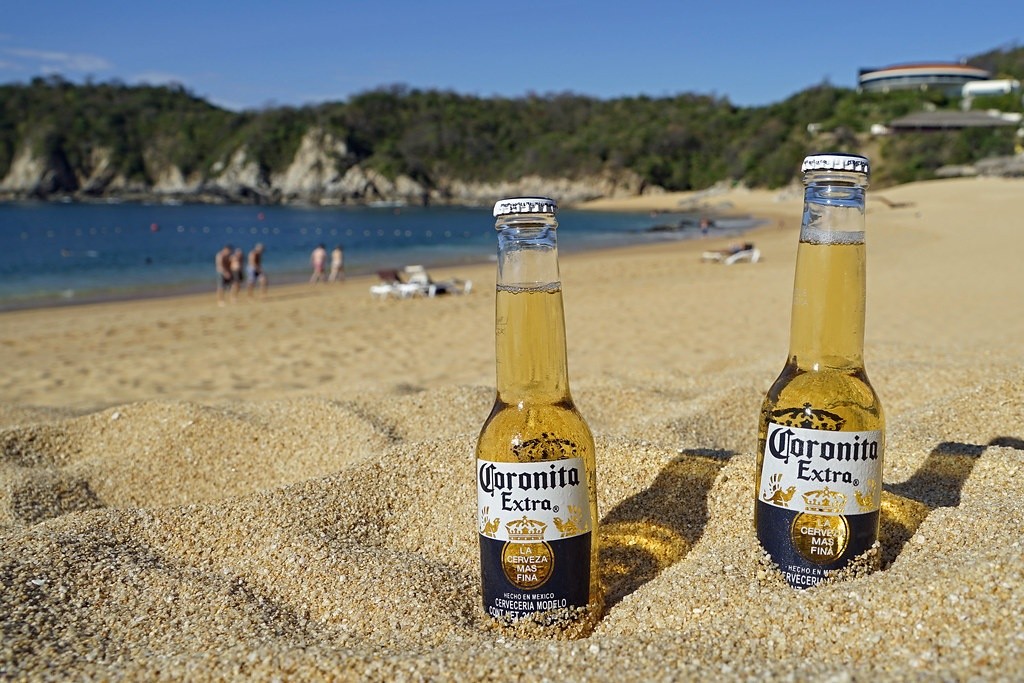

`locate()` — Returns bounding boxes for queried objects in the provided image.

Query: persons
[309,245,347,286]
[215,244,270,307]
[707,242,753,264]
[700,218,710,239]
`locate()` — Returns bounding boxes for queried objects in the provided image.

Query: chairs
[368,264,473,301]
[700,248,761,266]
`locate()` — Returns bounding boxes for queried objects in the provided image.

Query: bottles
[475,198,598,624]
[754,155,886,593]
